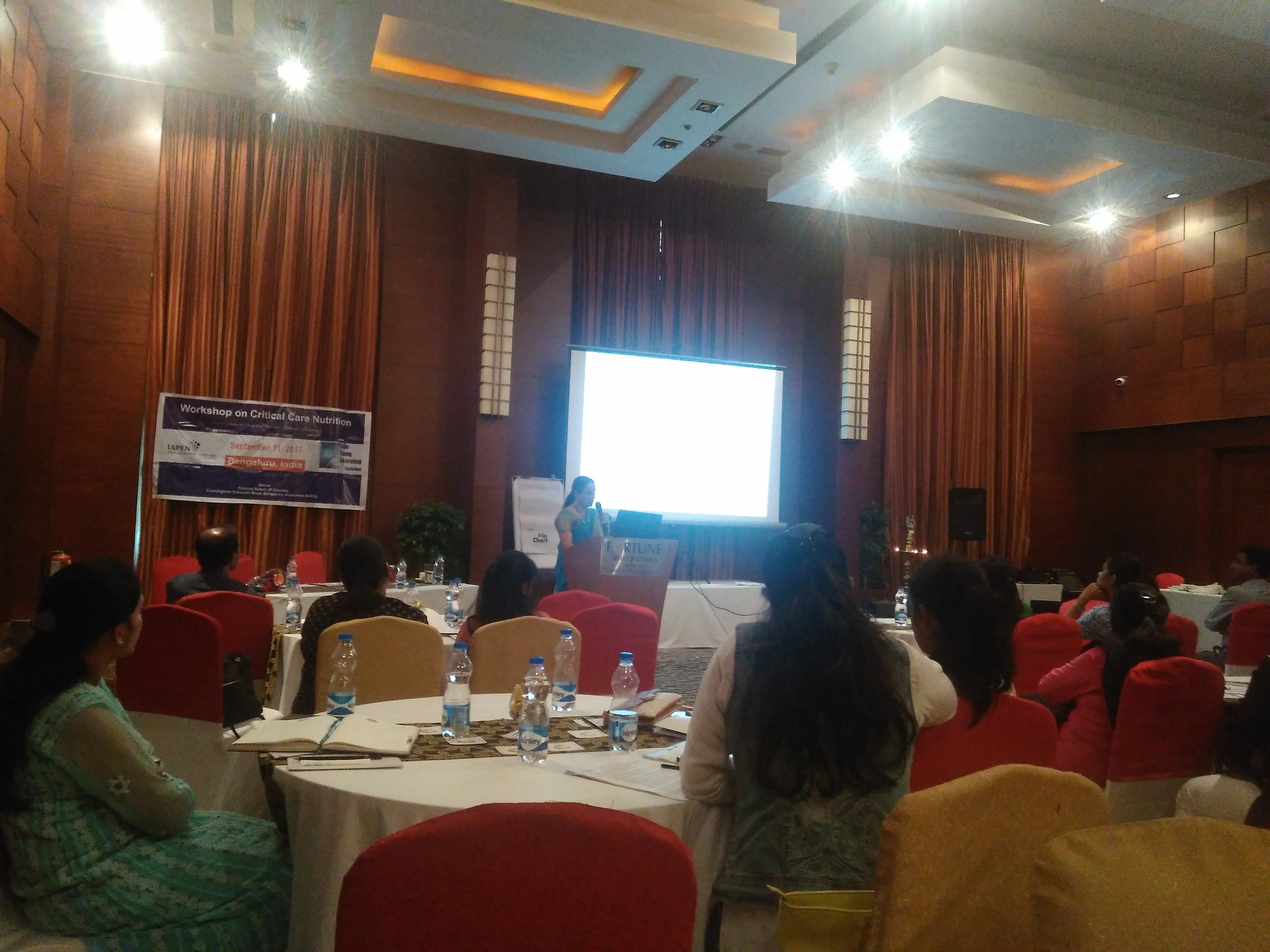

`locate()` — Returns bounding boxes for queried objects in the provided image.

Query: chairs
[332,801,699,952]
[1059,599,1110,645]
[1030,816,1270,952]
[152,551,660,717]
[1154,573,1185,590]
[1223,602,1269,677]
[1010,613,1084,698]
[907,691,1059,794]
[1104,658,1226,823]
[864,763,1119,952]
[105,604,282,822]
[1162,612,1199,658]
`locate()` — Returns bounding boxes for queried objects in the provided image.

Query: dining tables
[1221,674,1253,706]
[869,618,930,657]
[272,692,735,952]
[1160,588,1224,654]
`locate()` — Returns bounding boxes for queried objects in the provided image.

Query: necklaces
[574,505,590,526]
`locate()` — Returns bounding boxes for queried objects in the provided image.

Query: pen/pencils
[319,716,343,746]
[661,764,679,770]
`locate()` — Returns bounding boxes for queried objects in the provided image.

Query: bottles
[517,657,550,763]
[608,651,639,753]
[396,558,407,589]
[433,554,445,584]
[550,628,577,711]
[286,579,303,631]
[441,643,473,740]
[444,580,460,626]
[894,587,908,628]
[327,633,357,719]
[403,582,418,607]
[286,556,297,579]
[454,578,464,619]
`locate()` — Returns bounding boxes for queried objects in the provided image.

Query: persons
[291,537,431,715]
[1036,582,1189,793]
[978,551,1034,629]
[1171,697,1263,829]
[1194,548,1270,672]
[679,524,959,950]
[1064,553,1158,645]
[0,558,294,950]
[903,554,1017,788]
[1248,545,1270,580]
[453,549,550,647]
[552,476,615,594]
[1243,655,1270,832]
[165,526,260,606]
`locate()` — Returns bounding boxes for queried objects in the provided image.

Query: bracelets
[1073,602,1084,613]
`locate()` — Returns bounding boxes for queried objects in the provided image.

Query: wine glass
[509,687,526,738]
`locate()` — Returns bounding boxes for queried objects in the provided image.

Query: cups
[419,572,425,579]
[423,564,435,584]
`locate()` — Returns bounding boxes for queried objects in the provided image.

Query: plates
[414,578,425,581]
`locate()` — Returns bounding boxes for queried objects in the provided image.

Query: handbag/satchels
[766,882,874,952]
[220,652,264,729]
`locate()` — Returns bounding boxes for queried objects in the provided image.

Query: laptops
[609,509,662,538]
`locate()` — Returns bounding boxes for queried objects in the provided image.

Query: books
[227,714,421,755]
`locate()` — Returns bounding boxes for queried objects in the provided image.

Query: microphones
[595,501,608,534]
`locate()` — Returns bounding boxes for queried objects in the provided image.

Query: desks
[564,536,679,633]
[658,579,773,650]
[1014,583,1064,607]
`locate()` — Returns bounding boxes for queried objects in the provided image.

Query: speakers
[1050,568,1085,597]
[948,486,986,541]
